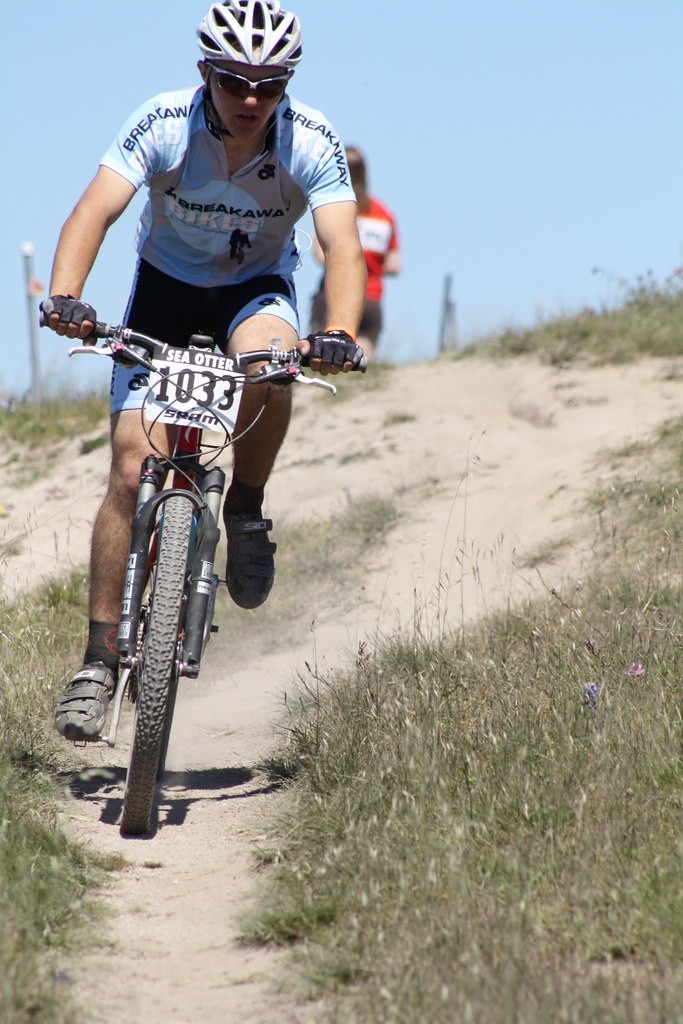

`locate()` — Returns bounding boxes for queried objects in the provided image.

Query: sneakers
[224,495,277,610]
[55,661,118,738]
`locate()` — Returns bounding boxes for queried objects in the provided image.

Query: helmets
[197,0,303,68]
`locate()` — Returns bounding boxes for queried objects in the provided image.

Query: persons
[40,0,368,739]
[309,144,399,365]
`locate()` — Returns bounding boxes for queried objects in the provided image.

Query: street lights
[20,238,44,415]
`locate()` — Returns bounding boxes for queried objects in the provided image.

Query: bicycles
[35,309,367,839]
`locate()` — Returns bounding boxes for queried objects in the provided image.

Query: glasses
[204,61,289,98]
